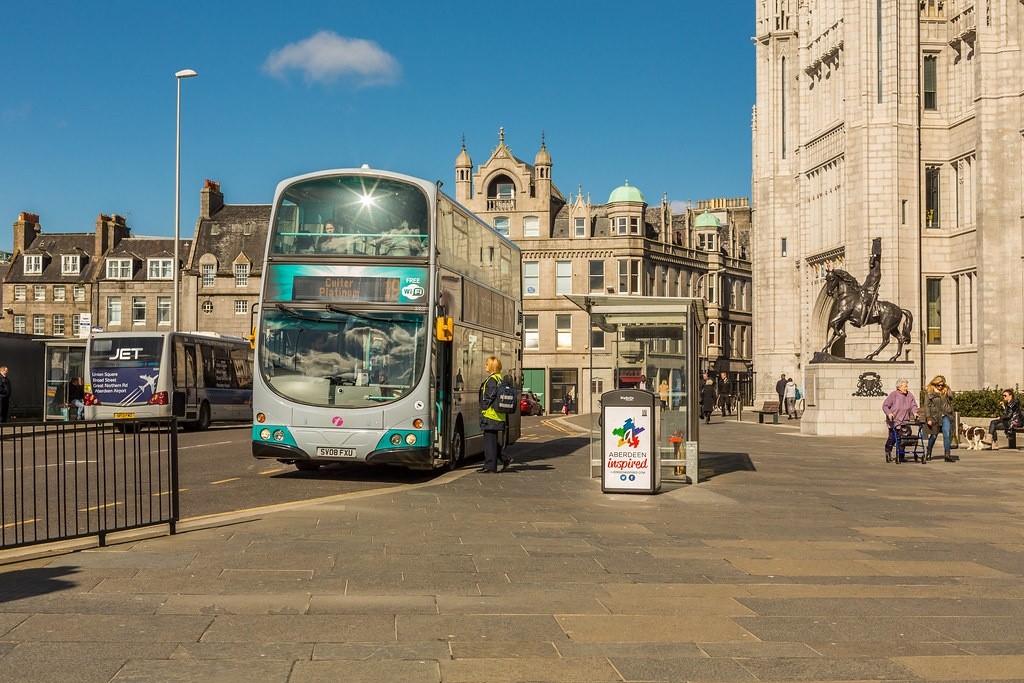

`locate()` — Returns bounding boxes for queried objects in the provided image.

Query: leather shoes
[477,467,497,473]
[502,457,514,471]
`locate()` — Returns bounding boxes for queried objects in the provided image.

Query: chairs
[291,230,315,253]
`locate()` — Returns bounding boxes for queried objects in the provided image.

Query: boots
[992,440,999,450]
[981,434,993,445]
[926,446,932,461]
[944,450,955,462]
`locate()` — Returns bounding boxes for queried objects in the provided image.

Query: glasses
[937,384,944,387]
[1003,395,1006,397]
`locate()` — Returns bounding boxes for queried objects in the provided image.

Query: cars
[521,392,543,416]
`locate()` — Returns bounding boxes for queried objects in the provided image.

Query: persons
[717,373,732,417]
[701,373,717,424]
[314,219,347,254]
[477,357,513,473]
[309,335,323,351]
[850,261,880,328]
[565,392,573,411]
[0,366,11,423]
[504,369,513,383]
[776,374,788,415]
[637,375,651,390]
[53,378,64,415]
[882,379,920,463]
[981,389,1024,450]
[785,378,798,419]
[382,222,422,256]
[924,376,955,462]
[659,380,668,408]
[69,377,84,420]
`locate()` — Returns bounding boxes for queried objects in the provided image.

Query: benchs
[960,417,1024,448]
[668,434,686,474]
[751,401,779,424]
[60,402,76,409]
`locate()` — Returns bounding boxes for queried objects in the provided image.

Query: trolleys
[892,416,927,464]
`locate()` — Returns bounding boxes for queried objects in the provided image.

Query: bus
[248,164,527,479]
[83,331,254,433]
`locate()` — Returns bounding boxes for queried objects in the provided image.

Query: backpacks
[490,375,517,414]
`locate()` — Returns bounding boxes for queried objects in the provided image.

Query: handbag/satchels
[795,384,800,400]
[699,406,704,419]
[561,405,568,414]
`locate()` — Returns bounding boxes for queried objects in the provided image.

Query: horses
[820,268,913,361]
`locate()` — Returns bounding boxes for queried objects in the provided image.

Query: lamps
[4,307,11,312]
[606,286,614,291]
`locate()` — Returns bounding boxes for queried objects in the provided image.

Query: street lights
[173,68,199,333]
[696,267,727,297]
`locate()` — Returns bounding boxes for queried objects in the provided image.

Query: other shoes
[885,451,897,463]
[663,408,666,412]
[660,408,662,412]
[899,451,908,462]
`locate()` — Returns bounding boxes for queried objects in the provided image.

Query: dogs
[959,423,988,451]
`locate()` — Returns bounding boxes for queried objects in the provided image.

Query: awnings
[620,377,641,382]
